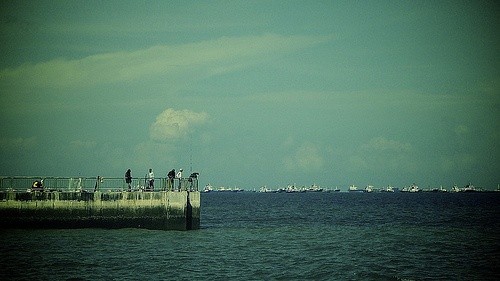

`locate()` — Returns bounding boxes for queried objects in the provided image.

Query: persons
[176,168,184,191]
[148,168,155,192]
[167,168,176,191]
[125,169,132,191]
[32,179,43,188]
[188,172,200,192]
